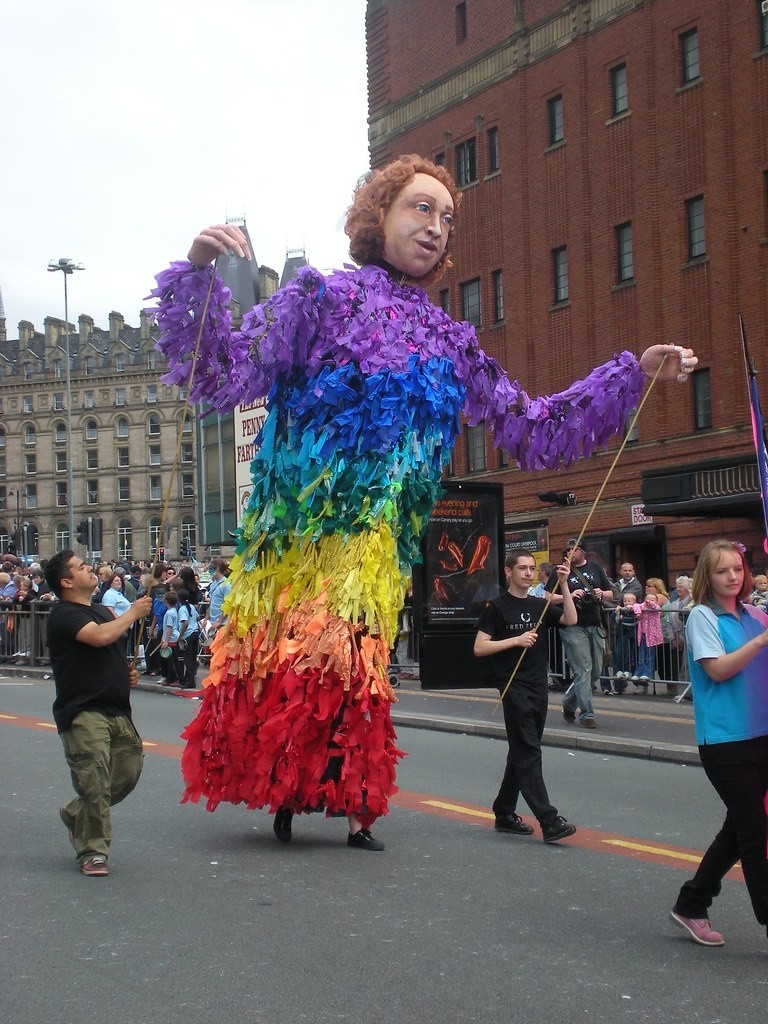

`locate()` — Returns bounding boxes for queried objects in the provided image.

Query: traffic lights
[179,538,187,556]
[75,521,88,545]
[9,529,20,549]
[159,548,164,562]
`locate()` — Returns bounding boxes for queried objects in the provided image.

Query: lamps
[537,491,575,507]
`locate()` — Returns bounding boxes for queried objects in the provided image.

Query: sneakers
[273,805,295,841]
[59,807,78,851]
[78,857,109,876]
[348,828,384,851]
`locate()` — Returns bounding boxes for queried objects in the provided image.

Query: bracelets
[600,592,605,602]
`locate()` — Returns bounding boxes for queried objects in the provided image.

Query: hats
[565,538,587,553]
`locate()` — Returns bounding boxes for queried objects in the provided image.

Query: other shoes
[562,707,576,724]
[142,669,196,689]
[539,817,577,842]
[668,908,724,946]
[579,714,598,729]
[494,811,535,836]
[602,669,651,696]
[12,650,31,658]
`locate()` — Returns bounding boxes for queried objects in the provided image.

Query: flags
[736,313,768,553]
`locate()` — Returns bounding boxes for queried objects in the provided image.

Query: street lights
[8,485,29,555]
[46,257,85,553]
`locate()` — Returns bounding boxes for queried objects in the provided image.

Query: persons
[39,551,153,877]
[472,544,578,843]
[670,537,768,947]
[535,522,768,727]
[0,550,235,691]
[152,149,697,852]
